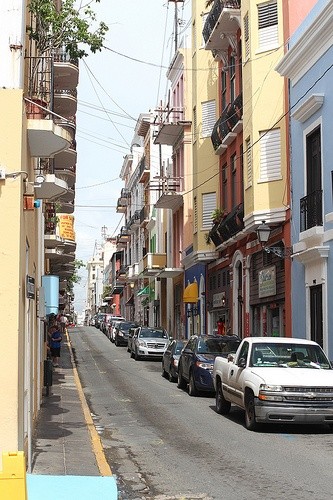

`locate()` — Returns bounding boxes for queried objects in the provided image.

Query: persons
[48,325,62,366]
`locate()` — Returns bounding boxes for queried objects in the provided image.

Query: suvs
[178,333,244,397]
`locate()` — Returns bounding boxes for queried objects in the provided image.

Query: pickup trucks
[211,337,333,431]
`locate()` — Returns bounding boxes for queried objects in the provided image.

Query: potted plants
[211,207,230,225]
[25,0,109,119]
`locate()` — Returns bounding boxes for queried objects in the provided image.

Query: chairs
[291,351,305,363]
[254,350,264,364]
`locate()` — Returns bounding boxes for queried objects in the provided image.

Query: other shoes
[53,362,59,366]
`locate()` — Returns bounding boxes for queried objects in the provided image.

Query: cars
[88,313,174,360]
[161,336,188,382]
